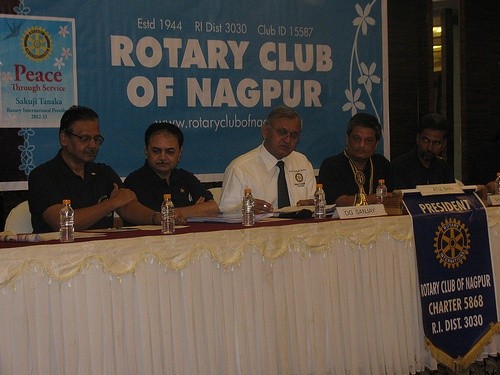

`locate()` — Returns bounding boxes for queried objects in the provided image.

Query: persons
[318,113,402,207]
[391,113,500,194]
[28,106,187,234]
[122,122,220,226]
[218,106,317,214]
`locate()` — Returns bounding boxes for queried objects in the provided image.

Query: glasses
[270,125,300,140]
[68,132,104,145]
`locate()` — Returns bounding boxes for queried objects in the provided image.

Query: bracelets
[152,213,157,224]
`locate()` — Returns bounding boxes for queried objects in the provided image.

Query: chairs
[4,200,33,233]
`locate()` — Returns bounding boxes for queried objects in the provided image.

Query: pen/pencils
[264,205,274,209]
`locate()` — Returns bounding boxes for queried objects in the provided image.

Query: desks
[0,194,500,375]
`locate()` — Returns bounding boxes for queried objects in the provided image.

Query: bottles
[242,189,255,226]
[376,179,387,203]
[314,184,327,219]
[59,200,74,242]
[161,194,176,234]
[495,173,500,195]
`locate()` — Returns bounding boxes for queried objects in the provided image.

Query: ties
[276,161,290,209]
